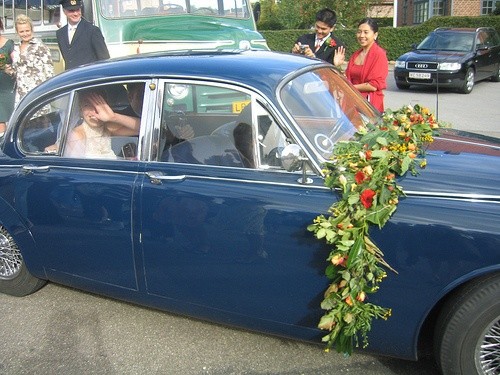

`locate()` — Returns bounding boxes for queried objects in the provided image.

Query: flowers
[0,49,9,66]
[322,35,337,48]
[304,103,452,358]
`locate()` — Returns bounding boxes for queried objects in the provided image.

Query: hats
[59,0,83,10]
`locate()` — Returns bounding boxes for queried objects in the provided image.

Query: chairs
[436,37,471,49]
[165,122,241,168]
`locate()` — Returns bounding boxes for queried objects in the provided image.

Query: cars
[0,48,500,375]
[392,26,500,94]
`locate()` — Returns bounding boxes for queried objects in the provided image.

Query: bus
[57,0,275,118]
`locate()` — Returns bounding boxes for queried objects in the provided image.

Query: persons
[333,17,389,113]
[56,0,110,71]
[0,19,17,138]
[11,14,56,101]
[290,9,347,66]
[64,83,194,161]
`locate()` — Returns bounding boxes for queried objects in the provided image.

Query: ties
[69,27,74,44]
[313,40,321,52]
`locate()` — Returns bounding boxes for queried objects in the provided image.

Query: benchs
[28,121,58,148]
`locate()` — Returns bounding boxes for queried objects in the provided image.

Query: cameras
[299,45,309,52]
